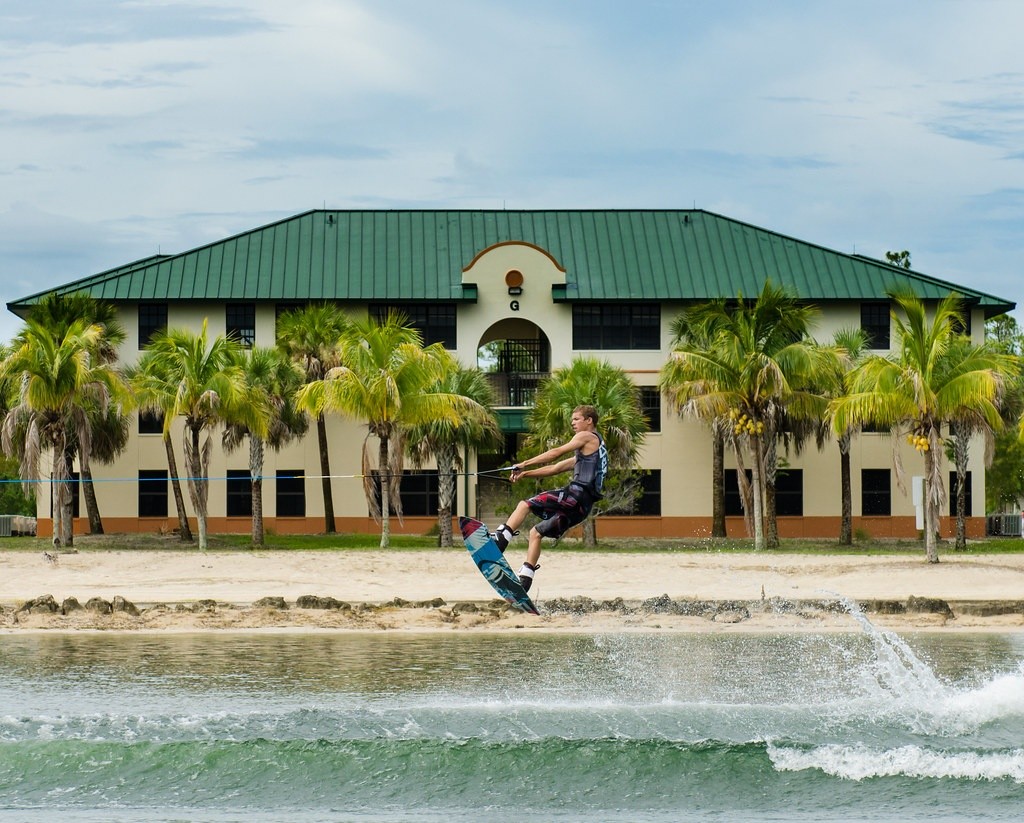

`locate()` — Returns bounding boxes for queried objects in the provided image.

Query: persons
[490,406,608,592]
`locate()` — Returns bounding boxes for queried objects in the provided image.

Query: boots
[515,562,540,593]
[489,524,520,553]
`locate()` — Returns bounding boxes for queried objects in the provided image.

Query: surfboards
[457,515,541,617]
